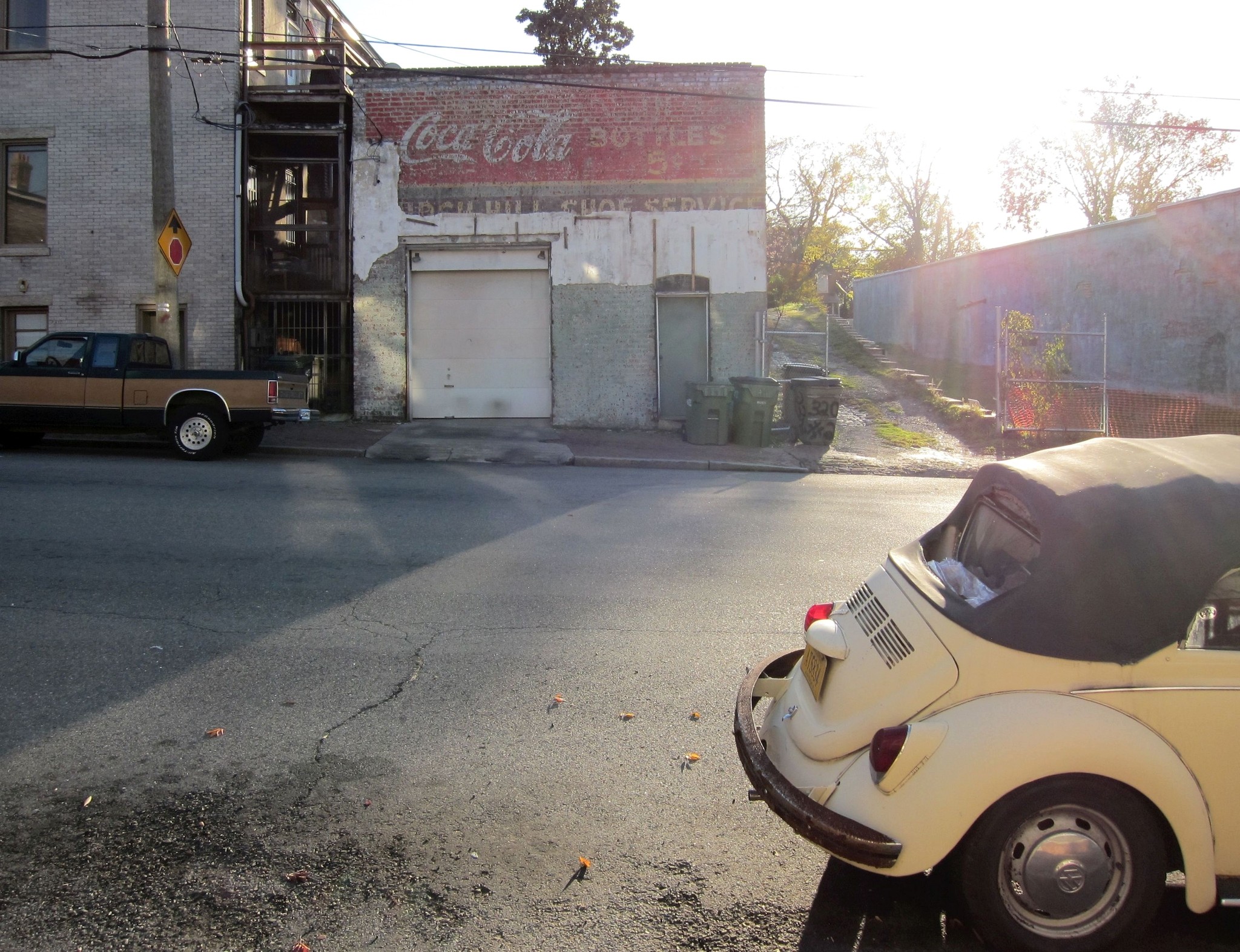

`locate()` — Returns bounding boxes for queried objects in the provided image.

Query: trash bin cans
[791,376,843,445]
[729,376,781,448]
[781,363,830,420]
[681,382,733,446]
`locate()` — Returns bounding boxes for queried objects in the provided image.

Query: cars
[734,430,1239,952]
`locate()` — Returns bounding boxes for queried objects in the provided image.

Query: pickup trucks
[0,329,313,461]
